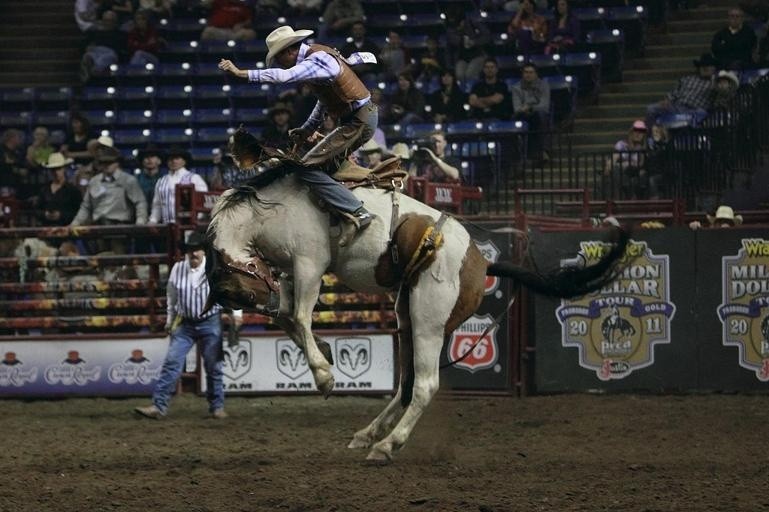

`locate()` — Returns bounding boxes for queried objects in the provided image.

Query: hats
[87,136,121,163]
[705,205,743,227]
[267,100,292,118]
[138,143,191,166]
[179,229,207,251]
[265,26,314,67]
[713,69,740,90]
[629,120,650,131]
[42,154,74,168]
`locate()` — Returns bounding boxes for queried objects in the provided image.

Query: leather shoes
[136,403,167,421]
[210,407,229,420]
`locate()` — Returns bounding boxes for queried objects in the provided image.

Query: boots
[339,205,372,246]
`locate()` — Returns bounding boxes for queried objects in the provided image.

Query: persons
[1,2,769,260]
[135,235,226,421]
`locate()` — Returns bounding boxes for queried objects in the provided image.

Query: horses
[198,157,643,467]
